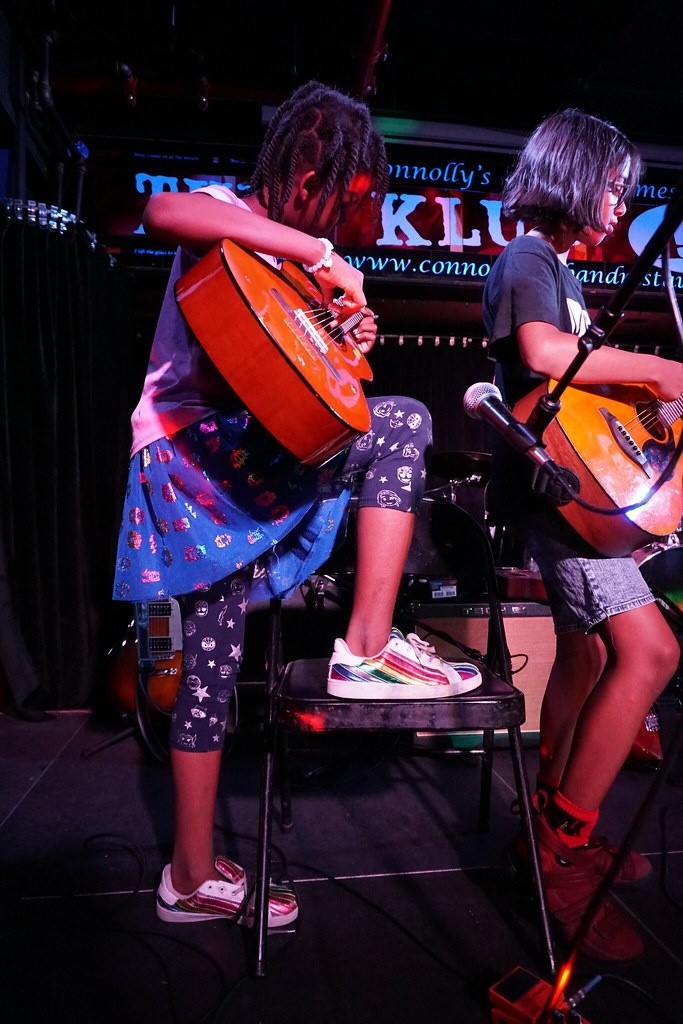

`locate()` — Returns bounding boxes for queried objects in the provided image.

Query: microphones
[462,382,563,484]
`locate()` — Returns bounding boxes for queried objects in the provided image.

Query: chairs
[256,499,562,983]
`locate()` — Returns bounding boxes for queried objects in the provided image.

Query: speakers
[390,599,558,752]
[237,572,345,675]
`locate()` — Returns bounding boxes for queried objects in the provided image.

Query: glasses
[603,177,632,202]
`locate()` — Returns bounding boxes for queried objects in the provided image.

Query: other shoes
[522,788,654,967]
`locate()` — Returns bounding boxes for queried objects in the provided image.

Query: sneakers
[156,856,300,929]
[327,627,483,699]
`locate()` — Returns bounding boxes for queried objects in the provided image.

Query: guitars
[107,598,184,719]
[510,365,683,559]
[173,237,374,468]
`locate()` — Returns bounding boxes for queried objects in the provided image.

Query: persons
[115,85,483,929]
[482,110,683,959]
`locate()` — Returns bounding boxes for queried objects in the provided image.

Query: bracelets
[303,236,338,276]
